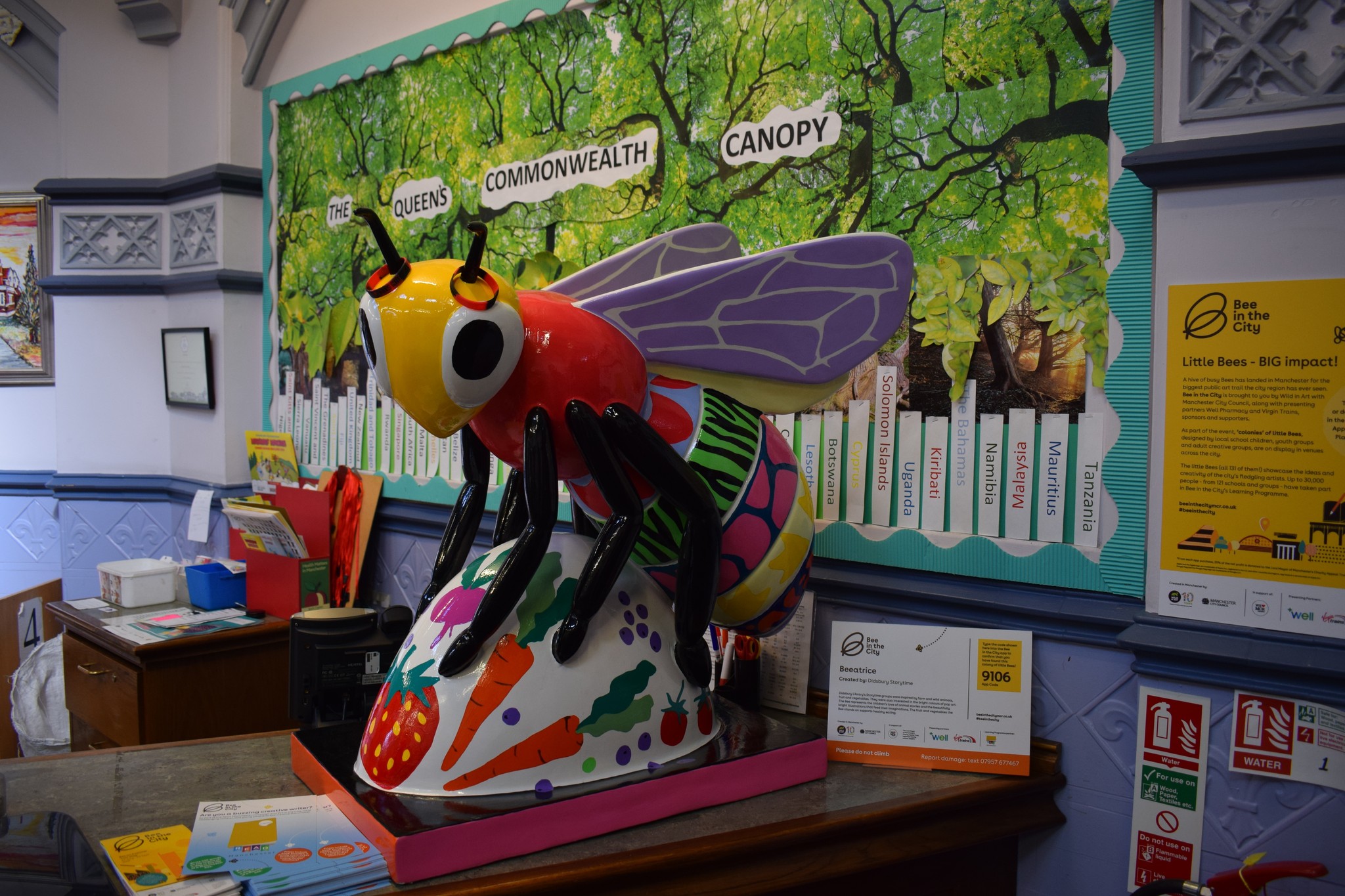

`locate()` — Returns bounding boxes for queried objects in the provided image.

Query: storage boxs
[175,560,247,612]
[97,558,178,609]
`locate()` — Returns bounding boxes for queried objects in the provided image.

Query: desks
[0,730,1068,896]
[43,595,296,748]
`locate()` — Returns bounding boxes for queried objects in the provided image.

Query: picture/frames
[161,327,216,409]
[0,191,56,387]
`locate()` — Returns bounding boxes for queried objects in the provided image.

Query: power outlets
[372,590,390,609]
